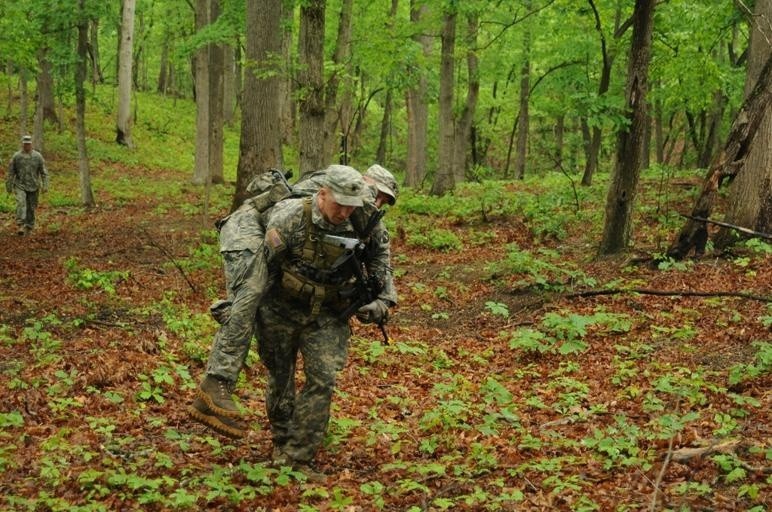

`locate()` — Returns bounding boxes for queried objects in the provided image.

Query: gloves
[355,299,390,324]
[210,299,233,324]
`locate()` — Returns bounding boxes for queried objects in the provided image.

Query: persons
[186,162,402,437]
[5,136,48,235]
[206,163,392,472]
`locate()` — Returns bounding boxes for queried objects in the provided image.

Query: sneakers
[18,226,32,236]
[187,376,249,439]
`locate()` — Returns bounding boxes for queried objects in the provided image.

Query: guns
[331,248,390,342]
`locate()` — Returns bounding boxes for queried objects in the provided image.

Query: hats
[20,135,32,143]
[323,163,364,208]
[365,164,400,206]
